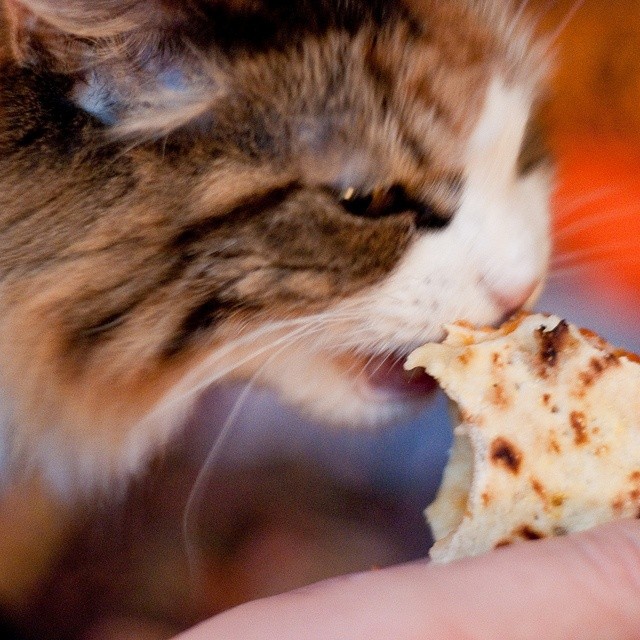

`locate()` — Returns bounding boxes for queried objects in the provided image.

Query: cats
[1,3,628,554]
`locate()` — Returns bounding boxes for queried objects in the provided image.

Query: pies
[404,312,640,567]
